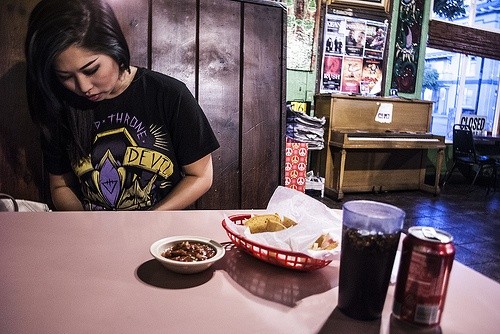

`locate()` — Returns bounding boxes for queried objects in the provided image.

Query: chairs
[442,124,497,195]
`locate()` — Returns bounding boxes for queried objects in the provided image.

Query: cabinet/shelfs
[0,0,287,209]
[313,93,434,193]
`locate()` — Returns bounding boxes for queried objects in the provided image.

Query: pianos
[313,93,447,201]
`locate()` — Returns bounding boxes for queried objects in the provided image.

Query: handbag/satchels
[1,194,53,212]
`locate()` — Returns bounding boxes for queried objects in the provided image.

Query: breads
[244,214,338,250]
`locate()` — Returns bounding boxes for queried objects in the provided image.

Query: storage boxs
[222,214,333,273]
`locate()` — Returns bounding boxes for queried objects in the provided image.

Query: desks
[0,209,500,334]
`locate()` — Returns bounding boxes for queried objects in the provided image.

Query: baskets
[222,213,334,271]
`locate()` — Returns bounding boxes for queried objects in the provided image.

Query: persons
[326,37,342,53]
[24,0,220,211]
[345,29,365,47]
[365,29,385,50]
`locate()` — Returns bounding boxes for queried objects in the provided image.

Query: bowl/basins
[149,235,225,274]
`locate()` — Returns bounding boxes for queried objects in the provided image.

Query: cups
[337,200,405,322]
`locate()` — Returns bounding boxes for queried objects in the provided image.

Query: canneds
[392,226,455,327]
